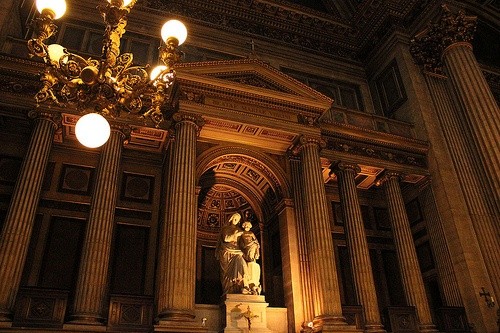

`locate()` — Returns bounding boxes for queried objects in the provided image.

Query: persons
[214,211,257,295]
[238,221,260,262]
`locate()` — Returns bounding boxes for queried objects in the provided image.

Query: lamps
[26,0,189,148]
